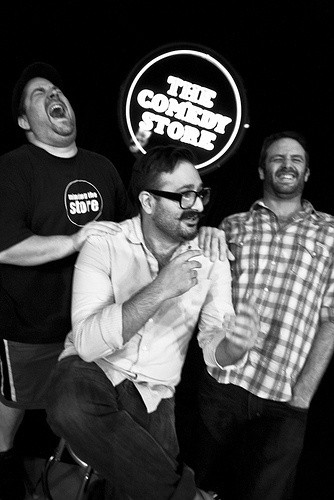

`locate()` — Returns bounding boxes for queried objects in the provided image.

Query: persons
[46,137,269,500]
[3,73,126,465]
[206,135,332,497]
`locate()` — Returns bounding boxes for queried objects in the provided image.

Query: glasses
[143,187,211,209]
